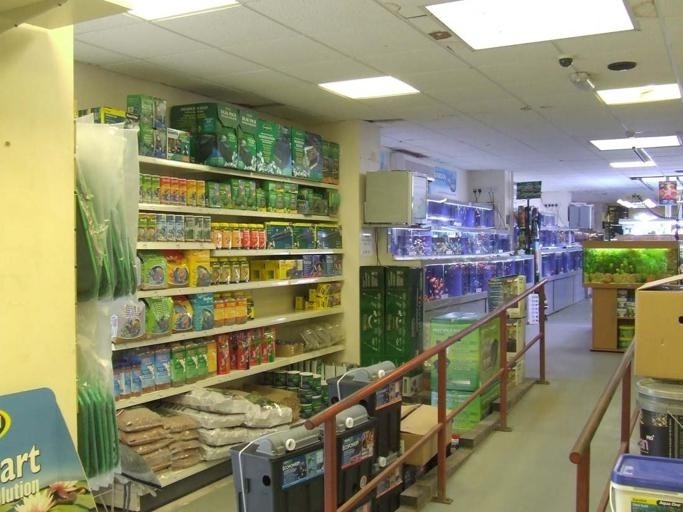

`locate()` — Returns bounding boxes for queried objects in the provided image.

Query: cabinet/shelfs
[592,287,632,352]
[75,154,348,512]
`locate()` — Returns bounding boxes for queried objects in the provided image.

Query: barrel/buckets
[636,378,683,460]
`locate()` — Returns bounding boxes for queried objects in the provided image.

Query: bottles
[224,293,235,326]
[313,374,322,395]
[214,294,224,328]
[265,371,273,385]
[257,224,266,249]
[221,223,231,249]
[239,224,251,249]
[249,224,259,249]
[230,223,241,249]
[297,388,312,403]
[235,293,247,324]
[247,295,254,321]
[219,258,230,284]
[322,383,329,409]
[257,372,266,384]
[273,370,286,387]
[229,257,240,282]
[211,223,222,250]
[239,257,249,282]
[299,372,314,389]
[313,395,322,414]
[286,370,299,387]
[299,404,313,419]
[210,258,220,285]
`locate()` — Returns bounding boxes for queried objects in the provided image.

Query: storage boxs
[313,410,380,497]
[430,383,500,429]
[320,140,341,183]
[171,101,239,166]
[608,453,682,512]
[504,316,526,361]
[237,108,259,173]
[430,313,500,392]
[507,360,524,385]
[231,428,332,512]
[398,404,453,467]
[633,273,682,383]
[291,125,323,182]
[257,117,292,177]
[488,274,526,318]
[324,357,400,458]
